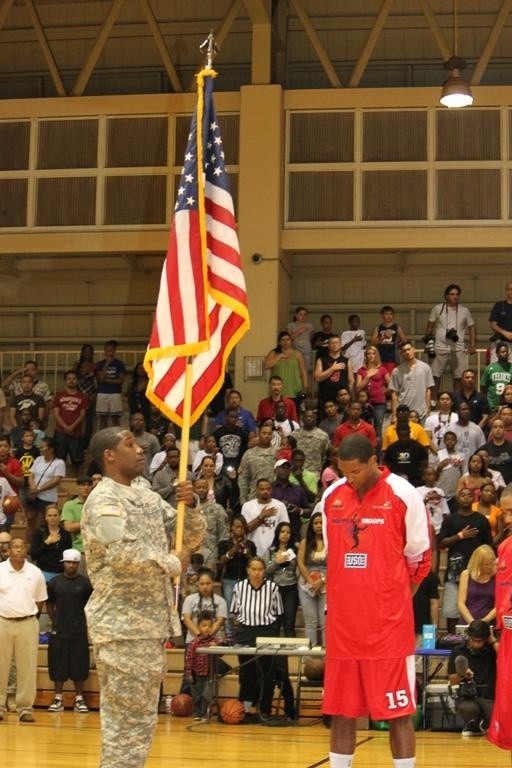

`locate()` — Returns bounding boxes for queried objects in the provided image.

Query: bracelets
[225,552,234,559]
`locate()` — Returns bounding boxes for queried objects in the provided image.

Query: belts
[0,615,35,621]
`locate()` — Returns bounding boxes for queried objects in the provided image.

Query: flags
[143,31,250,425]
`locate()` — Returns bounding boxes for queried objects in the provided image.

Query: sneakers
[286,714,296,723]
[462,723,482,737]
[261,713,273,722]
[74,699,89,713]
[48,699,64,712]
[20,711,35,724]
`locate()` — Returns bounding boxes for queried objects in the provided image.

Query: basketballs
[219,700,247,725]
[170,692,193,714]
[304,655,325,680]
[3,496,20,514]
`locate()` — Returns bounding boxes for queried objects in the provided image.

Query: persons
[379,404,442,481]
[0,387,7,434]
[471,483,504,547]
[81,426,208,768]
[192,480,229,557]
[289,449,318,524]
[185,610,225,723]
[129,410,161,483]
[74,344,98,452]
[457,545,498,630]
[219,516,258,640]
[183,553,210,593]
[299,513,331,649]
[213,407,249,497]
[0,477,24,531]
[474,448,507,490]
[128,361,152,433]
[231,557,284,727]
[13,428,40,488]
[290,409,329,495]
[91,470,102,489]
[276,435,297,461]
[436,432,464,494]
[1,361,53,430]
[0,434,25,495]
[193,434,224,476]
[241,478,294,562]
[29,418,45,449]
[455,454,496,506]
[48,550,92,712]
[9,408,33,449]
[31,505,71,644]
[238,425,277,505]
[180,569,228,721]
[60,475,91,550]
[250,418,283,438]
[29,437,66,508]
[148,433,176,475]
[448,620,501,737]
[319,444,341,489]
[10,375,46,427]
[154,449,194,507]
[196,456,235,508]
[332,404,377,454]
[0,538,48,723]
[323,434,432,768]
[415,466,450,534]
[474,419,512,484]
[262,523,300,638]
[270,458,313,545]
[486,482,512,751]
[51,371,90,477]
[94,340,127,430]
[258,282,512,420]
[213,390,256,435]
[440,489,493,640]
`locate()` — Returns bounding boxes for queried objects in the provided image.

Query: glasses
[399,411,410,416]
[448,293,460,296]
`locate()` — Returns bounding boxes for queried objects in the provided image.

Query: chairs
[296,656,323,722]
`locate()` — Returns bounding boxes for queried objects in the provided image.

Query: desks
[414,649,451,730]
[194,645,326,724]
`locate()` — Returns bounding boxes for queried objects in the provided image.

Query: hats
[59,549,81,562]
[272,459,291,471]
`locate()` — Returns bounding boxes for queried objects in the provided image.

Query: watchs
[458,532,465,541]
[257,516,265,523]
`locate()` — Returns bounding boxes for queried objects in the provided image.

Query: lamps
[440,0,473,107]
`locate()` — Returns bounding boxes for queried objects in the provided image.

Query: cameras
[423,334,437,359]
[446,327,459,343]
